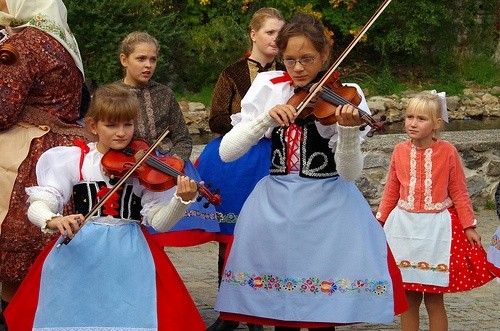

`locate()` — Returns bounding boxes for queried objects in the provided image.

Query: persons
[375,90,495,331]
[214,8,410,331]
[0,0,91,331]
[4,84,207,331]
[113,32,220,247]
[207,8,287,331]
[487,182,500,282]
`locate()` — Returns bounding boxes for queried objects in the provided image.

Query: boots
[247,322,264,331]
[207,256,239,331]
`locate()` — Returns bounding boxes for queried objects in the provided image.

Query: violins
[101,138,222,209]
[287,71,386,138]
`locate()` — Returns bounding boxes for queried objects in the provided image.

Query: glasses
[281,55,320,67]
